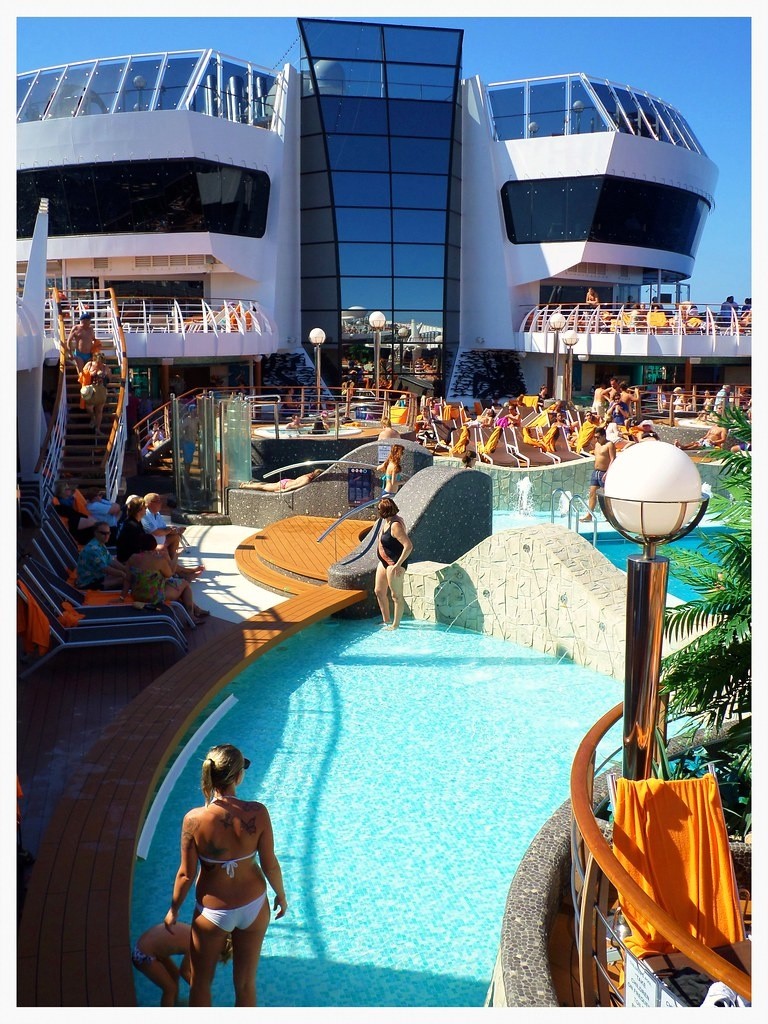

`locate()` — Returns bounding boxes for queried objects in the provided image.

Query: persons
[180,404,199,473]
[342,355,437,406]
[579,428,616,521]
[374,498,413,631]
[206,375,250,395]
[416,389,459,447]
[342,326,357,334]
[238,468,325,492]
[285,387,323,412]
[462,450,477,470]
[647,367,663,382]
[286,410,330,434]
[67,312,113,436]
[464,383,579,453]
[107,388,165,447]
[54,481,210,626]
[377,416,405,499]
[567,287,751,337]
[132,744,287,1007]
[586,384,752,453]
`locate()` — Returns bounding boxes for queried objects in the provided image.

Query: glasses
[244,757,251,771]
[614,398,620,400]
[98,531,111,535]
[152,499,163,504]
[594,436,603,439]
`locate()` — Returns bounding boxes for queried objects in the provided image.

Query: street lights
[433,335,444,379]
[368,309,387,402]
[397,327,408,376]
[592,438,713,788]
[307,327,326,415]
[559,329,582,409]
[548,310,566,401]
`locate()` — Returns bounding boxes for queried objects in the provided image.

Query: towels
[443,405,465,424]
[613,772,747,991]
[447,426,470,457]
[390,406,408,424]
[523,426,557,453]
[610,311,632,332]
[522,395,539,413]
[576,421,599,455]
[484,426,502,454]
[686,318,704,328]
[647,312,667,327]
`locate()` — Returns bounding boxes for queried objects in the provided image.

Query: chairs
[17,479,197,679]
[525,306,752,336]
[414,396,660,468]
[146,302,234,331]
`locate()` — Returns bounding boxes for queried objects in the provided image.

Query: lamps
[528,122,540,138]
[132,76,148,109]
[572,100,585,133]
